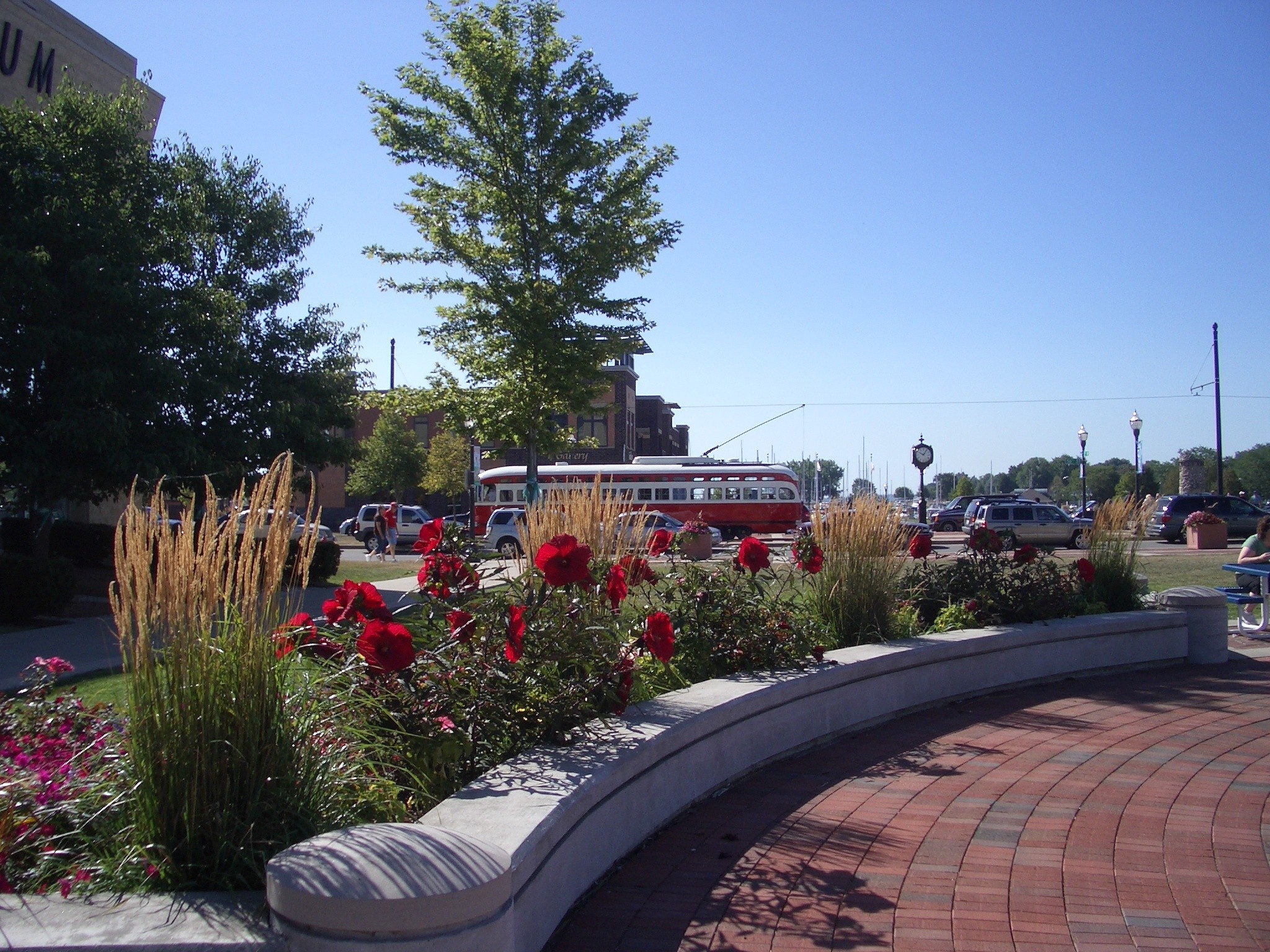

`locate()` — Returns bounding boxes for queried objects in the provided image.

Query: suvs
[482,506,566,559]
[1146,492,1270,545]
[972,500,1097,552]
[351,503,467,554]
[928,493,1019,532]
[961,496,1054,536]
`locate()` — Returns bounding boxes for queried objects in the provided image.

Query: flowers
[1180,510,1227,527]
[681,510,713,535]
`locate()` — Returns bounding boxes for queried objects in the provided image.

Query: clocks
[911,444,933,472]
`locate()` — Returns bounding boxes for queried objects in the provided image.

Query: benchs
[1215,587,1251,593]
[1224,592,1264,605]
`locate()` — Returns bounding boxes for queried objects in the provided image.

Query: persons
[726,489,751,500]
[365,506,387,563]
[485,485,507,502]
[1235,515,1270,625]
[376,502,399,562]
[1034,497,1041,516]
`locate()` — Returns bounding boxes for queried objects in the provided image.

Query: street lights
[1130,408,1145,536]
[1078,423,1088,519]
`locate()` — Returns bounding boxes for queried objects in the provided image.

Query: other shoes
[392,559,397,563]
[365,554,372,562]
[376,554,382,561]
[1242,610,1258,625]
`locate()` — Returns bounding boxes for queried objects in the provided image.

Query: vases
[678,534,712,560]
[1186,523,1227,550]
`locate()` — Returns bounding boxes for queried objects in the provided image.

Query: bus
[465,404,806,542]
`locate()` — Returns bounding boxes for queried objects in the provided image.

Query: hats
[391,502,399,508]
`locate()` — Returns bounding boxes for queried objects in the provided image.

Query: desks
[1221,563,1270,639]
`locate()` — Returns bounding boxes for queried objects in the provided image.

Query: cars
[217,505,250,534]
[236,508,335,546]
[893,499,951,520]
[141,505,181,536]
[339,517,353,537]
[797,510,933,540]
[443,511,471,525]
[599,510,722,550]
